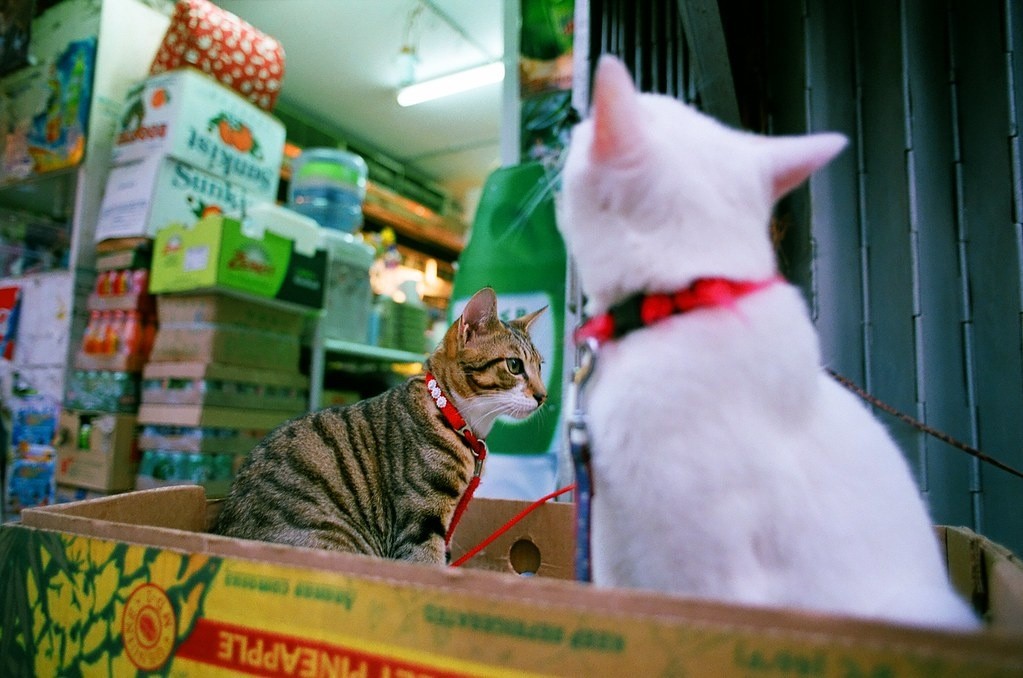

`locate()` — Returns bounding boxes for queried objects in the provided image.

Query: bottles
[289,148,366,233]
[359,228,395,255]
[83,310,156,355]
[379,280,427,353]
[67,371,138,412]
[140,426,263,499]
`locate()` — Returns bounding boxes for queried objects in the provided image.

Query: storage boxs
[0,485,1023,677]
[1,2,330,519]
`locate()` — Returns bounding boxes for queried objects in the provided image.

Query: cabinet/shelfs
[276,141,465,364]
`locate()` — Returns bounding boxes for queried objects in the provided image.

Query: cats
[551,52,986,635]
[207,285,551,566]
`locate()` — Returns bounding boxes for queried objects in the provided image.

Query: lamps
[388,0,507,108]
